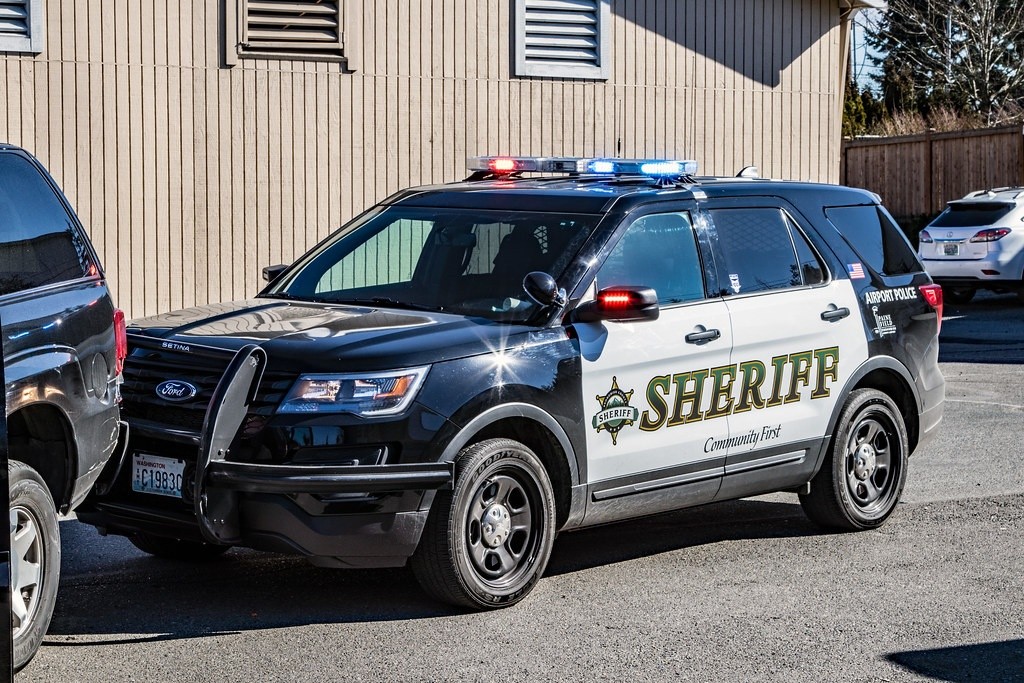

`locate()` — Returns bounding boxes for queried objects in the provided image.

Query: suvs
[73,155,950,614]
[2,142,132,675]
[918,185,1024,308]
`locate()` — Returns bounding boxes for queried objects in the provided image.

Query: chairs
[489,233,546,304]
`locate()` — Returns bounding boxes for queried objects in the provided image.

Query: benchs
[728,249,795,292]
